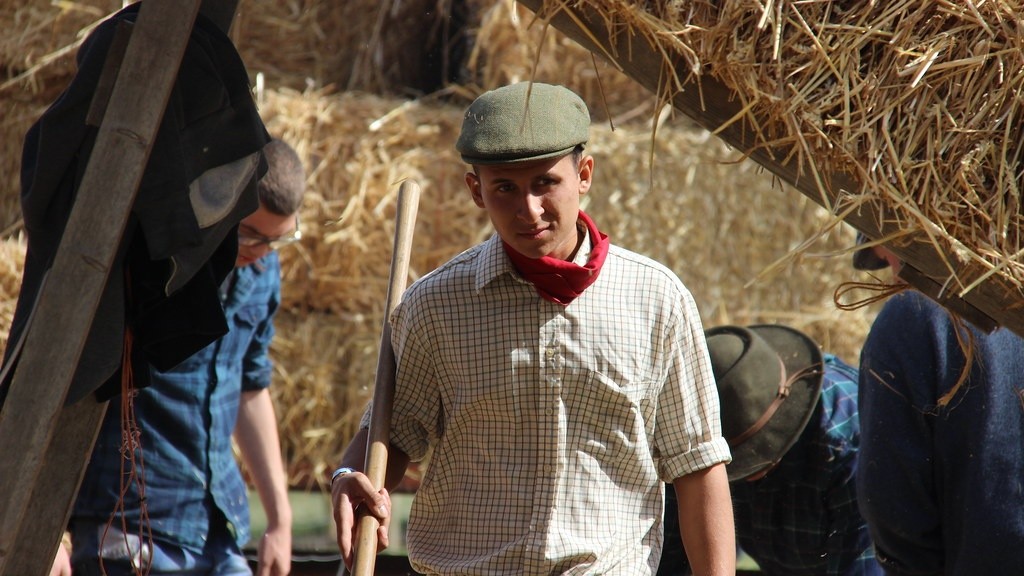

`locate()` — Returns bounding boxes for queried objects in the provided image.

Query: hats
[703,323,828,481]
[852,232,890,269]
[456,82,590,163]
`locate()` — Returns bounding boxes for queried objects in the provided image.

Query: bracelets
[332,467,355,480]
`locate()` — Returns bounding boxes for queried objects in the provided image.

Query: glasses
[236,214,303,249]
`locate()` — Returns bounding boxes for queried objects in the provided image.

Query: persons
[66,138,305,576]
[656,325,886,576]
[330,80,736,576]
[854,231,1024,576]
